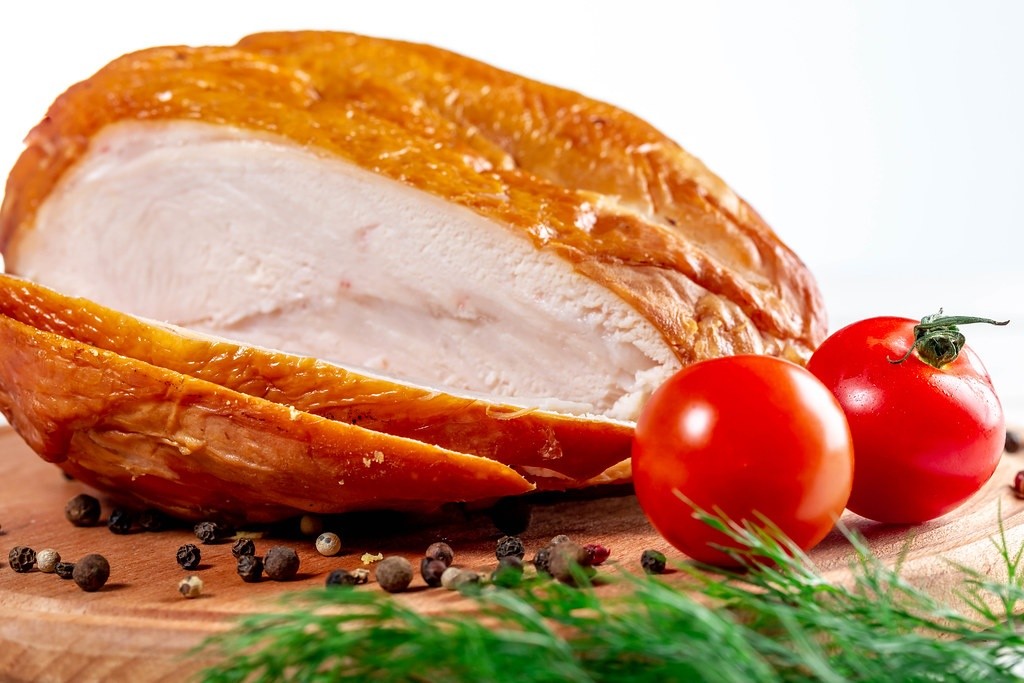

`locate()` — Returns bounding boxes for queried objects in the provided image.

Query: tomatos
[632,312,1009,569]
